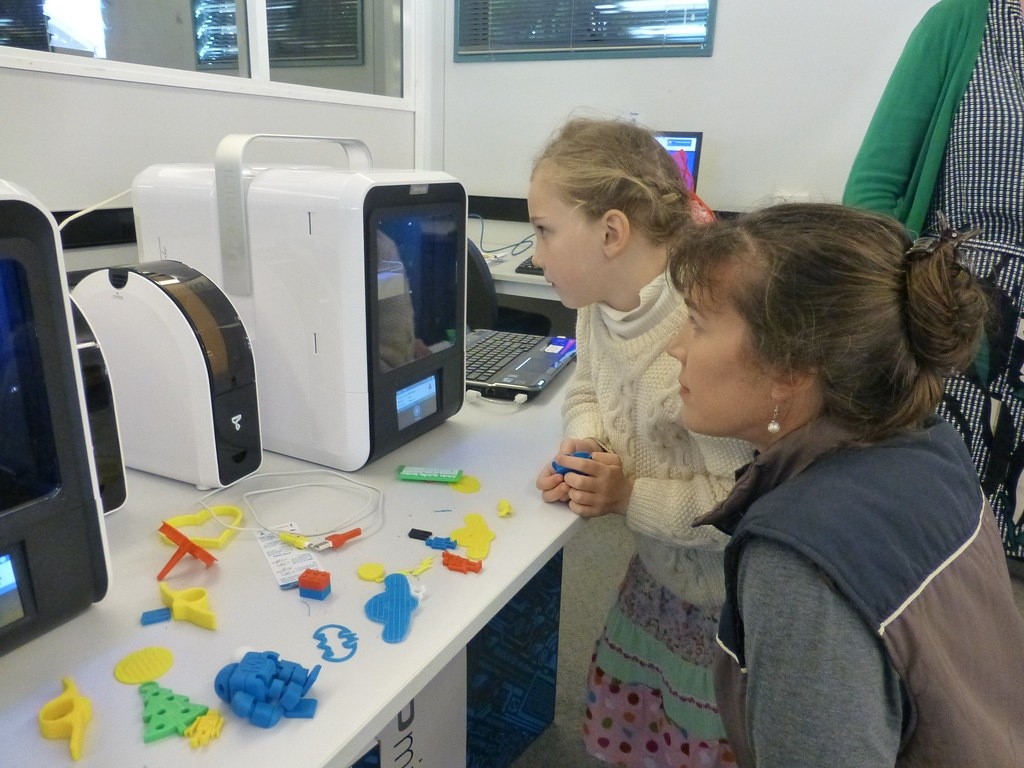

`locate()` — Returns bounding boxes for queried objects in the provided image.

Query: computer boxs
[0,179,109,658]
[129,135,468,472]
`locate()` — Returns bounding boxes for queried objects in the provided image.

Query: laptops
[465,324,577,401]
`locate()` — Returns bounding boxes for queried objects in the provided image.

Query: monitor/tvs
[652,132,702,196]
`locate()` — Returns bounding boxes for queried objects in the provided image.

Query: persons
[369,231,435,376]
[834,0,1024,598]
[668,202,1024,768]
[526,120,771,767]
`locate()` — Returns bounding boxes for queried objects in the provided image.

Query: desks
[0,352,591,768]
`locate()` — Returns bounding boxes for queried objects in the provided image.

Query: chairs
[467,238,550,337]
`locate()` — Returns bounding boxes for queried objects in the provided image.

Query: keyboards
[515,254,545,275]
